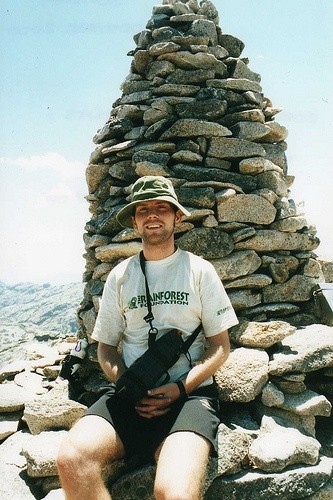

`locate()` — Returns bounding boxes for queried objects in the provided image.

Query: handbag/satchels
[103,330,187,424]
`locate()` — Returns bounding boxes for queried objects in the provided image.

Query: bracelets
[174,380,188,400]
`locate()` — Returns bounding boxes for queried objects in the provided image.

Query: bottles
[68,336,88,376]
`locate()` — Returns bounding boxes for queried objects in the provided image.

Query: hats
[116,176,191,229]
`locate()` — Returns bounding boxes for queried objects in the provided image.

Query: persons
[54,174,240,500]
